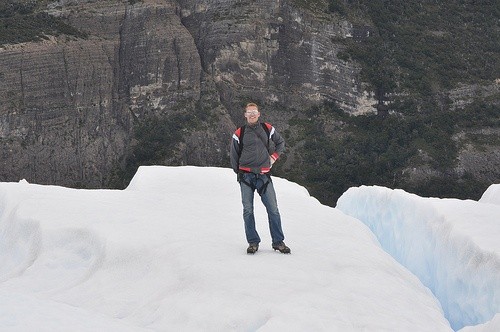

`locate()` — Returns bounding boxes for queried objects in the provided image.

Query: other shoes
[247,243,258,254]
[272,242,290,254]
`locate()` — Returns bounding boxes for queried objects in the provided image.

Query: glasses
[244,110,258,117]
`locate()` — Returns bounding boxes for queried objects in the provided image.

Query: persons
[230,103,291,254]
